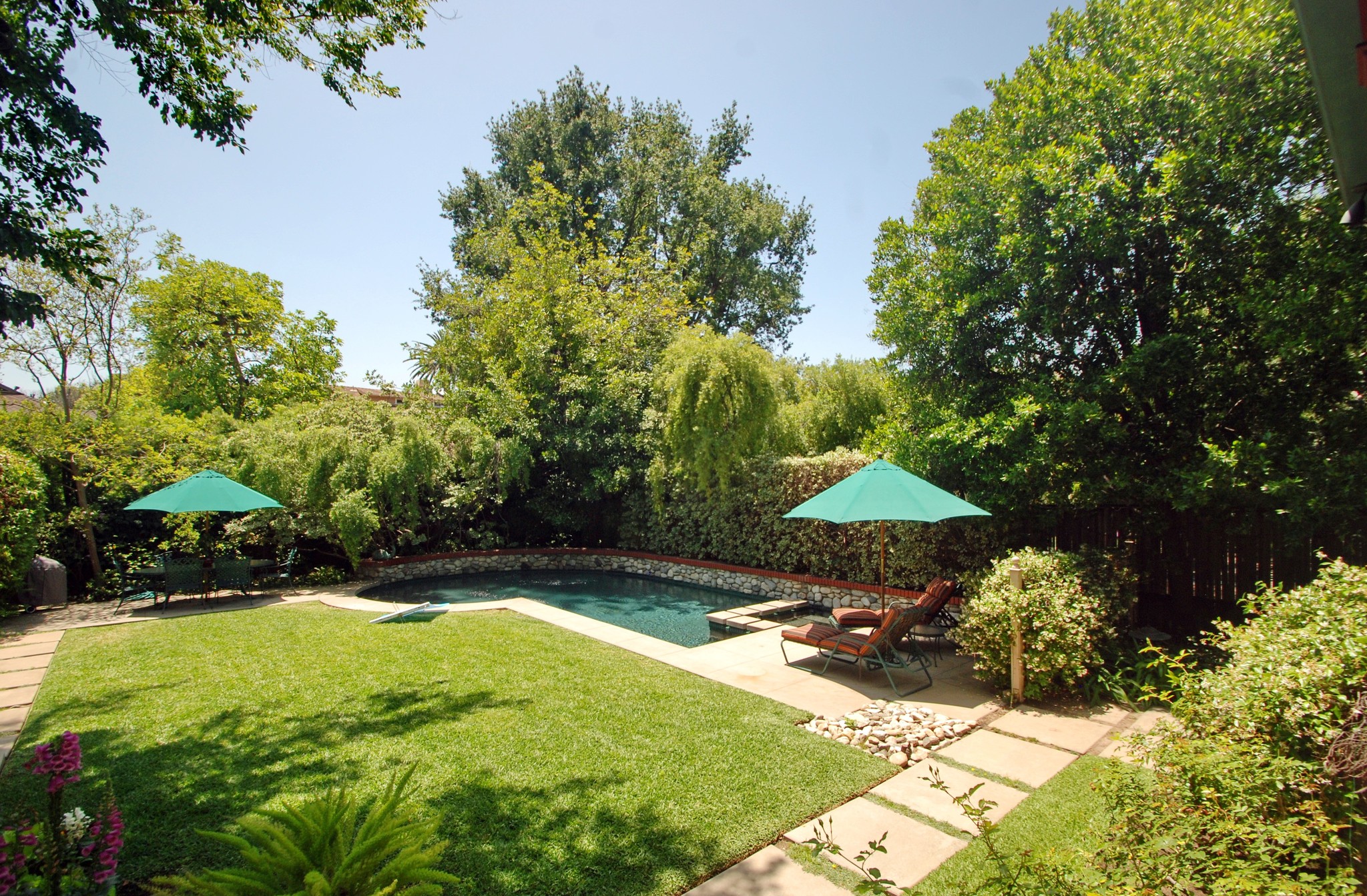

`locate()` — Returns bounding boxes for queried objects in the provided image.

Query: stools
[904,622,948,662]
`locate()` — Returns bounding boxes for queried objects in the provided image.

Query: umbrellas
[123,466,285,601]
[781,453,992,621]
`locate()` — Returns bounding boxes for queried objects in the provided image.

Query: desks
[131,558,275,608]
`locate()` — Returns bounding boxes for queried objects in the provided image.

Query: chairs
[201,549,247,597]
[110,555,161,615]
[154,551,199,603]
[258,546,300,599]
[828,575,962,659]
[212,555,256,607]
[161,551,208,613]
[779,603,934,697]
